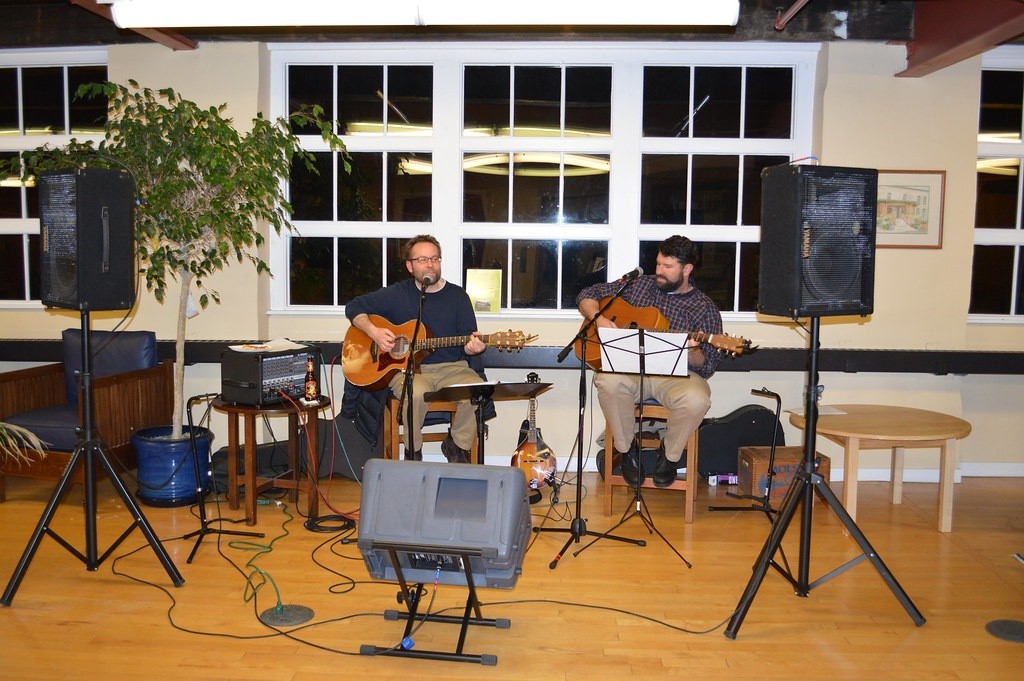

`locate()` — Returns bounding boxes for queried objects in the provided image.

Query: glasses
[408,256,442,264]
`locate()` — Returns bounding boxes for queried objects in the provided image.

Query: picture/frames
[875,169,947,250]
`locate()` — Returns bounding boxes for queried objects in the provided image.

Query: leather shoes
[404,448,423,461]
[441,429,469,464]
[621,435,646,487]
[653,438,678,486]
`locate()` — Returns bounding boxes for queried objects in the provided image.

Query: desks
[790,404,972,537]
[212,395,331,527]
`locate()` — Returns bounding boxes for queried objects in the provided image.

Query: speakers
[356,458,532,590]
[723,317,927,641]
[38,165,136,313]
[757,164,879,321]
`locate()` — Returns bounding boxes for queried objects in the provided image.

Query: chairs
[383,388,478,465]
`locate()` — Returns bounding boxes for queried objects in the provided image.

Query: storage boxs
[738,446,831,503]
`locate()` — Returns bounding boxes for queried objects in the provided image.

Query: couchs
[0,329,174,511]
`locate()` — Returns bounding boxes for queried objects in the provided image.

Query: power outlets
[260,604,314,627]
[986,619,1024,642]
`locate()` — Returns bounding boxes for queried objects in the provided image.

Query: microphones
[622,267,643,281]
[421,274,433,290]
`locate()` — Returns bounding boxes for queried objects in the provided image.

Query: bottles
[304,356,318,401]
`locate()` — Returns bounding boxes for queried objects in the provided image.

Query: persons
[576,236,724,487]
[345,236,486,464]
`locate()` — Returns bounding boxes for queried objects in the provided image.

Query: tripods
[0,311,186,607]
[182,393,265,564]
[531,282,704,569]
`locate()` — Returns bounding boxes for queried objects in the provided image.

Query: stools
[604,398,698,522]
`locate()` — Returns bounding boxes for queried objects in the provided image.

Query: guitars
[341,313,541,395]
[510,371,558,490]
[574,294,760,375]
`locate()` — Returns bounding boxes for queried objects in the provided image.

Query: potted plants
[0,76,354,508]
